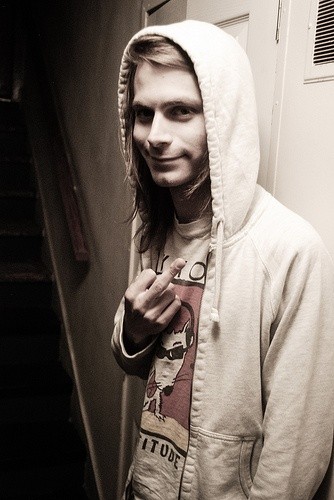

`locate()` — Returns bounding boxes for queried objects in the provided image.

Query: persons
[109,17,333,500]
